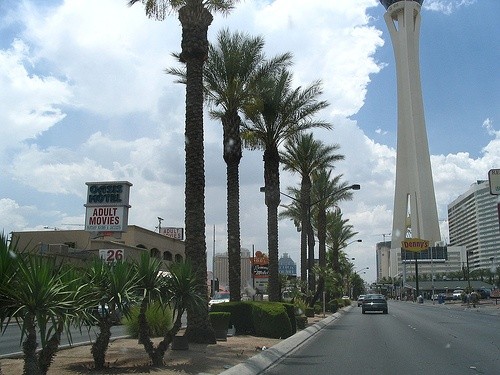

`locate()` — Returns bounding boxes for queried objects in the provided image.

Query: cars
[342,296,349,299]
[362,294,388,314]
[358,295,366,307]
[432,294,439,301]
[453,290,464,297]
[209,292,230,307]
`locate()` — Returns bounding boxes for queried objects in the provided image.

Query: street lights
[323,240,363,252]
[260,184,361,291]
[466,250,474,292]
